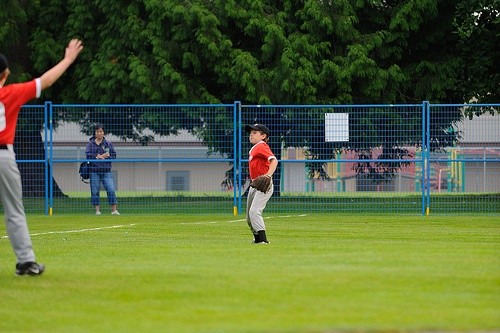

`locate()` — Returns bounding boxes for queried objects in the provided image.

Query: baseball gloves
[249,174,273,194]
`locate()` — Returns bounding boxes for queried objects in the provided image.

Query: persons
[85,126,120,215]
[0,38,83,277]
[244,124,278,245]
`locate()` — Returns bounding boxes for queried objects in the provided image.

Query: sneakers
[16,260,47,277]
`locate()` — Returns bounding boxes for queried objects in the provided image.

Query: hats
[0,54,11,75]
[244,123,270,136]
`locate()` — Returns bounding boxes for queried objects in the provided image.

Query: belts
[0,144,8,150]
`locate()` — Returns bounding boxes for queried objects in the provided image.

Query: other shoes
[112,210,121,215]
[96,211,100,215]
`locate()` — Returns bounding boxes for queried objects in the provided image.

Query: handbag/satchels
[79,162,90,184]
